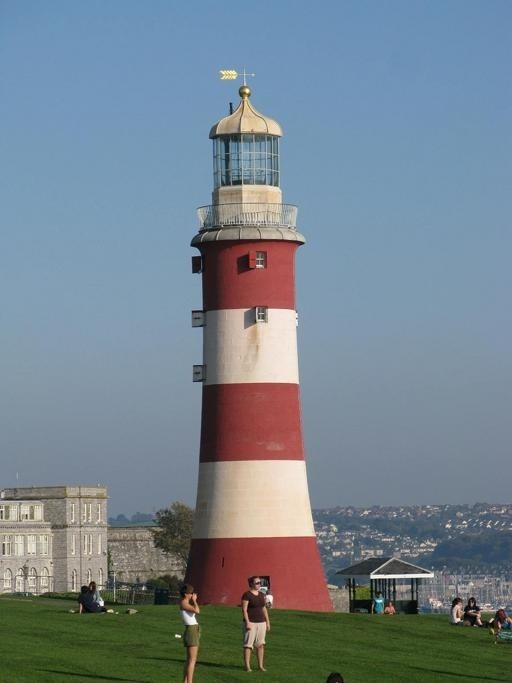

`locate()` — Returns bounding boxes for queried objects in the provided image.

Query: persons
[326,672,344,683]
[463,597,483,626]
[78,586,114,614]
[385,601,395,614]
[449,598,464,625]
[179,584,200,683]
[371,591,385,615]
[88,581,100,608]
[478,609,512,632]
[241,576,270,672]
